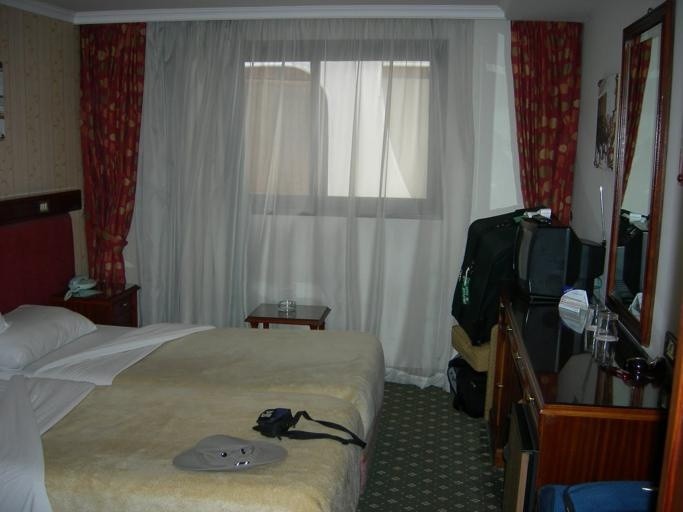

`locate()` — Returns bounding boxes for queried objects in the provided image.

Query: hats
[172,434,288,471]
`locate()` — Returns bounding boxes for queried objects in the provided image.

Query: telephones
[68,275,97,290]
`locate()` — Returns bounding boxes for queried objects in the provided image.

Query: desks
[242,300,331,331]
[490,290,673,511]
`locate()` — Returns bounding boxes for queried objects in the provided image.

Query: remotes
[532,214,552,225]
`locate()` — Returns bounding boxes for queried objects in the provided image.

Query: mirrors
[605,0,678,351]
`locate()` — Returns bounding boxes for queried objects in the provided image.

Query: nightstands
[66,281,142,330]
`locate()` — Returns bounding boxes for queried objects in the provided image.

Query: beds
[1,187,384,443]
[4,374,368,512]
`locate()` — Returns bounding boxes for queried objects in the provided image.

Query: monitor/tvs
[512,214,605,304]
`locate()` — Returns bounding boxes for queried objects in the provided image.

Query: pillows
[1,303,97,374]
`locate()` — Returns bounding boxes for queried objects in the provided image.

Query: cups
[277,290,296,312]
[593,312,619,342]
[588,304,608,331]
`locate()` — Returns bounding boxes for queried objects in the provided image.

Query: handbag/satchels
[533,479,657,512]
